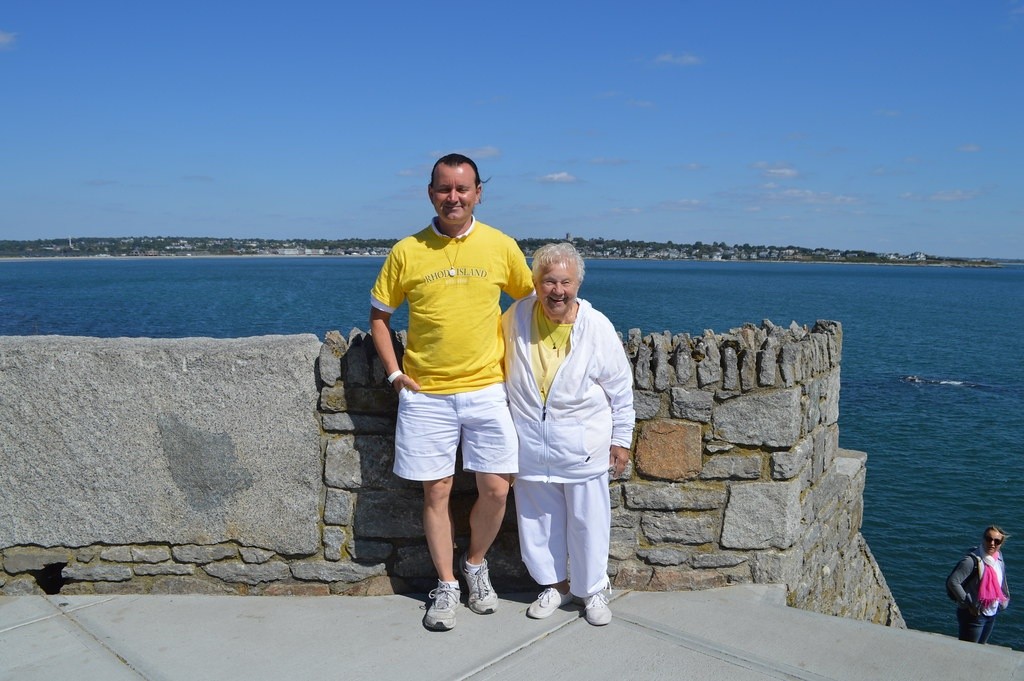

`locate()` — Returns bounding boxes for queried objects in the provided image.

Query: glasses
[984,535,1004,545]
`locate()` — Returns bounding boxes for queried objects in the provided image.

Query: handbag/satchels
[945,554,980,605]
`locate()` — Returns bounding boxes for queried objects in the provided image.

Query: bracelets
[388,370,402,383]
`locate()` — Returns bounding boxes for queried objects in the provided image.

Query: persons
[500,243,636,626]
[370,153,534,632]
[947,526,1010,644]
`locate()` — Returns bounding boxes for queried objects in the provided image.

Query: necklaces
[441,236,462,277]
[543,309,572,349]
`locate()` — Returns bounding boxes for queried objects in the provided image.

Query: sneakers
[527,587,574,619]
[424,578,461,631]
[583,591,612,626]
[462,559,498,615]
